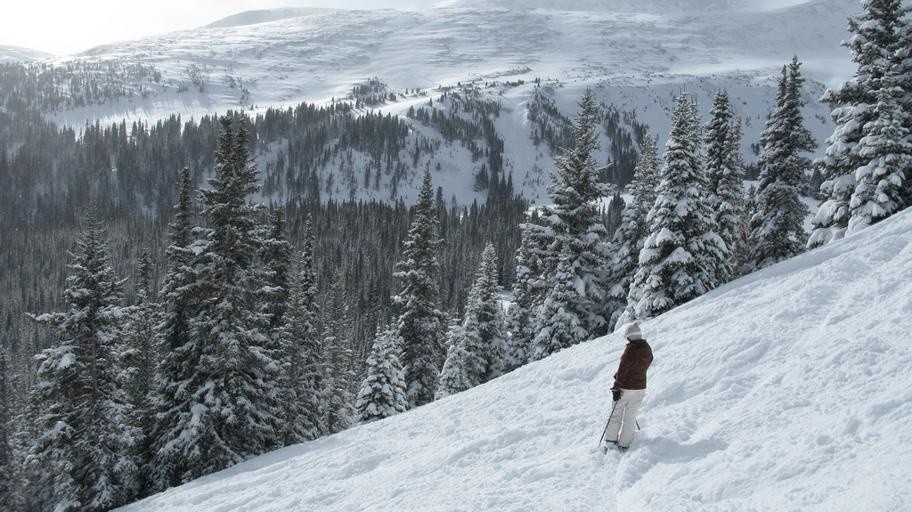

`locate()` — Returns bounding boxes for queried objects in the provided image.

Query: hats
[624,321,642,341]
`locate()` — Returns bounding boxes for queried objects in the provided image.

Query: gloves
[609,388,621,401]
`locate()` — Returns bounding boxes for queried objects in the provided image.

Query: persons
[605,323,654,451]
